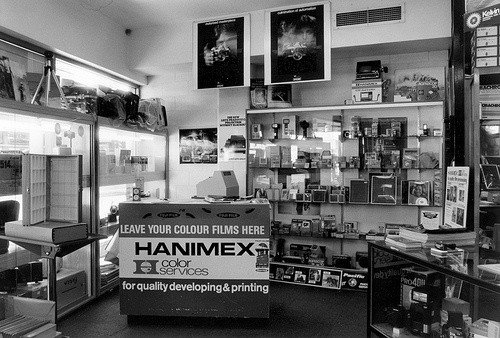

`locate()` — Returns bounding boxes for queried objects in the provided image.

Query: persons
[277,15,317,56]
[204,22,243,88]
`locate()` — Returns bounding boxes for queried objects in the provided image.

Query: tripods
[32,61,70,109]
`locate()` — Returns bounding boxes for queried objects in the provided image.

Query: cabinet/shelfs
[0,0,500,338]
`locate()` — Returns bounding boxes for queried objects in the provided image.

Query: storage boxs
[352,78,386,104]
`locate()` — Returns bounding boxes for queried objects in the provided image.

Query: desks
[0,232,108,332]
[367,239,500,338]
[119,197,271,327]
